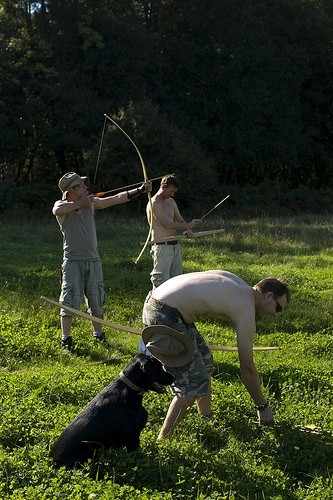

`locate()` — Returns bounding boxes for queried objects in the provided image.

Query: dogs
[48,353,177,481]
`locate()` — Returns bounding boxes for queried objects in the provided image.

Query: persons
[53,171,153,358]
[139,269,290,446]
[144,175,202,289]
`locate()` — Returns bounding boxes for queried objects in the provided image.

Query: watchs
[253,401,268,412]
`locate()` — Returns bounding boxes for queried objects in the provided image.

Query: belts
[151,240,178,245]
[146,294,184,323]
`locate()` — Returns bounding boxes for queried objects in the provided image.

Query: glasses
[273,295,283,313]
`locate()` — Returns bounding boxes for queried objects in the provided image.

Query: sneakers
[60,334,75,351]
[93,331,111,349]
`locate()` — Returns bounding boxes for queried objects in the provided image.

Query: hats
[58,172,89,200]
